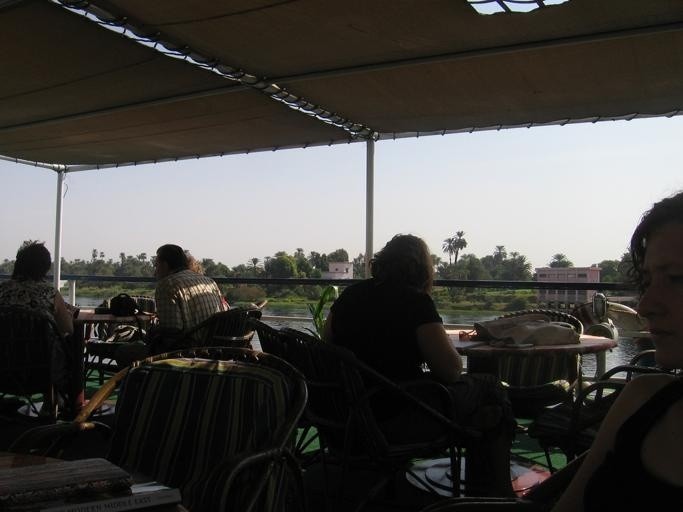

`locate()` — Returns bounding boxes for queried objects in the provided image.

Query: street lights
[473,313,580,347]
[110,293,140,317]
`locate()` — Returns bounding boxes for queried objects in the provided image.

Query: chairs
[1,299,683,511]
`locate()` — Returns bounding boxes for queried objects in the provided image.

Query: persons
[0,240,83,423]
[544,189,682,511]
[321,232,518,496]
[114,243,230,373]
